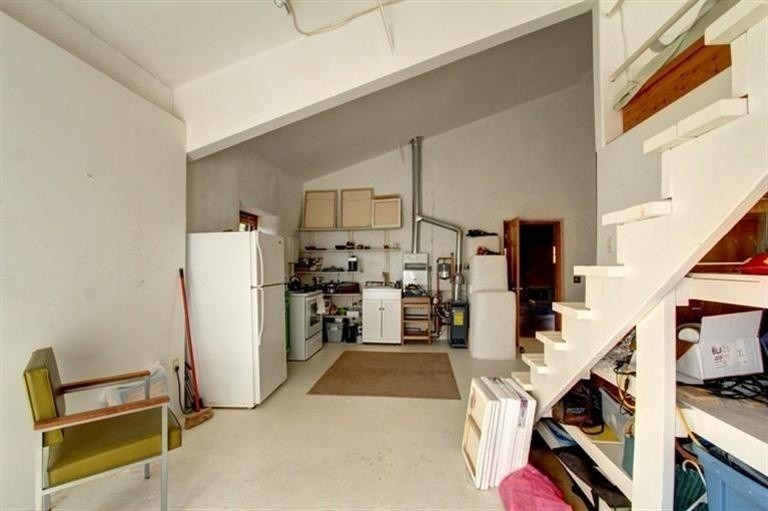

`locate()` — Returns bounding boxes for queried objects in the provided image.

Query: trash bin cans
[343,323,359,343]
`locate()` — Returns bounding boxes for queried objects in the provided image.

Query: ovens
[305,295,323,341]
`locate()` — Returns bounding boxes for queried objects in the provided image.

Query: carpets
[306,351,461,399]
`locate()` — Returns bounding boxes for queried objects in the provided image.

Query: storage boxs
[694,441,768,510]
[600,388,631,441]
[678,309,762,379]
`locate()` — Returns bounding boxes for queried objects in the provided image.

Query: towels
[317,295,326,314]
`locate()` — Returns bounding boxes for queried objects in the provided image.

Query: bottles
[348,255,357,271]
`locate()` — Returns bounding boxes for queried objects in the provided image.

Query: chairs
[22,348,182,511]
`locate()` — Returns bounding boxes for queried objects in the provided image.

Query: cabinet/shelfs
[294,228,400,317]
[537,274,768,510]
[362,289,401,344]
[401,296,431,345]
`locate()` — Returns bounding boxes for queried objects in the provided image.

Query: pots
[322,282,338,294]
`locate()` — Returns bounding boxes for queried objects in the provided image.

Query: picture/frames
[341,188,374,228]
[371,198,401,228]
[304,190,337,228]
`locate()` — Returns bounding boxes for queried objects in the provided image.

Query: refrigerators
[185,232,289,411]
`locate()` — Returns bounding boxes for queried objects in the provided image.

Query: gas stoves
[288,286,319,294]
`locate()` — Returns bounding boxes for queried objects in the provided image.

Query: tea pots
[287,276,302,291]
